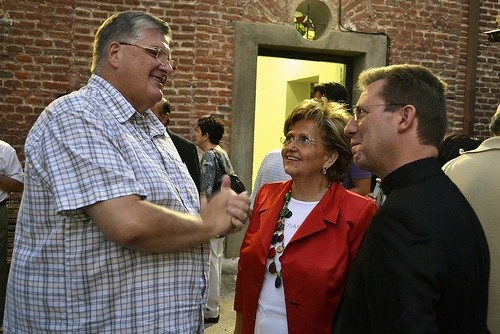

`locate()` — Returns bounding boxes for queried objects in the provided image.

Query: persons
[440,102,500,334]
[234,97,378,334]
[0,138,24,326]
[192,115,226,324]
[331,65,490,334]
[148,96,201,200]
[312,82,372,196]
[246,151,292,220]
[2,12,250,334]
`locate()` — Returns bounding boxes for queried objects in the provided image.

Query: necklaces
[267,188,292,288]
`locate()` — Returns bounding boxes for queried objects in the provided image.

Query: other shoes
[204,315,219,324]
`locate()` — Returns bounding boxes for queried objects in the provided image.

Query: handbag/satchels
[201,149,246,196]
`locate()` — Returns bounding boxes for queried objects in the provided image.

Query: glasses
[353,103,406,120]
[119,42,178,72]
[280,136,330,147]
